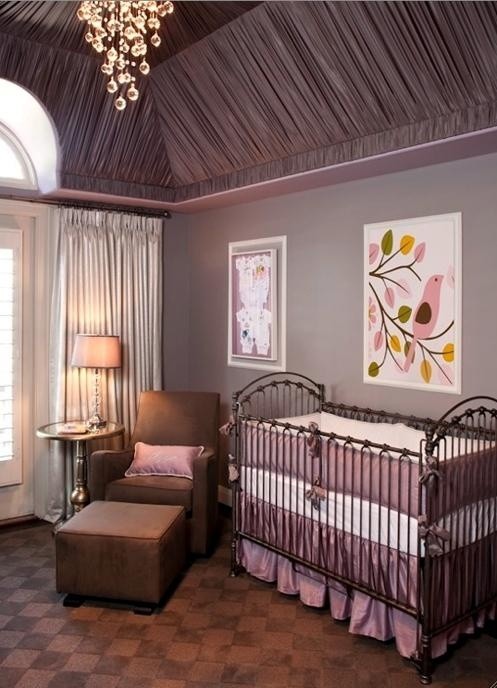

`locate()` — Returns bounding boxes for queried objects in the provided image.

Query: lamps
[70,334,122,426]
[76,0,174,112]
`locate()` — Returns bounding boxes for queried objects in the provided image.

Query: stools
[55,501,188,616]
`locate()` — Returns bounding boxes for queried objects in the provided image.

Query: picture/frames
[362,210,463,395]
[226,234,287,373]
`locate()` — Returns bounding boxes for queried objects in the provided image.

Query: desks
[36,420,125,535]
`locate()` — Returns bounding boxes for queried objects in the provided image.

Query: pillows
[123,441,204,482]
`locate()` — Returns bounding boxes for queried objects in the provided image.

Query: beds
[229,371,496,685]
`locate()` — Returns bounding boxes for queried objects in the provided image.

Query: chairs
[90,390,220,556]
